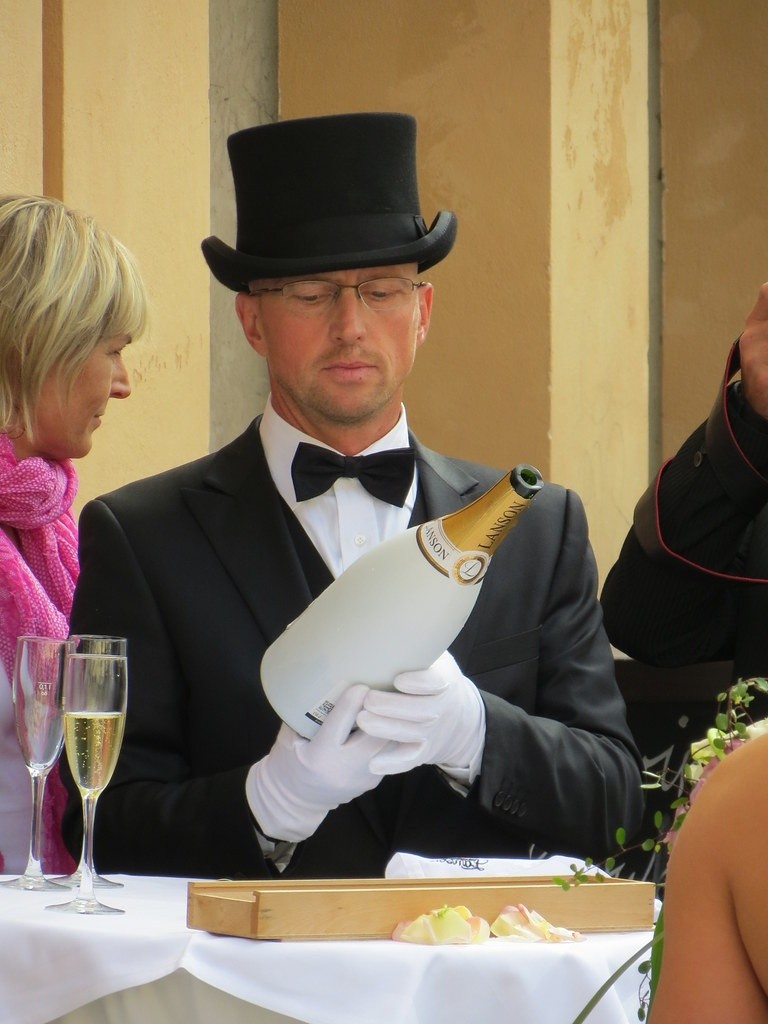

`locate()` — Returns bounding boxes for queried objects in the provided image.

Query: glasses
[247,274,423,310]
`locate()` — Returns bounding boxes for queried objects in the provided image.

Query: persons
[70,108,642,881]
[0,190,145,875]
[594,281,768,878]
[651,736,768,1023]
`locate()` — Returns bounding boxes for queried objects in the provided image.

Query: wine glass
[0,636,72,891]
[43,652,130,916]
[47,632,128,891]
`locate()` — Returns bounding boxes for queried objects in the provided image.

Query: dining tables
[0,874,663,1022]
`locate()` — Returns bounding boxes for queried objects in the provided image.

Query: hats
[200,113,458,292]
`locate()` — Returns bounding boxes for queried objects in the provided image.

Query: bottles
[260,463,542,740]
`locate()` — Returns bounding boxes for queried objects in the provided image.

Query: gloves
[246,683,387,848]
[361,653,487,776]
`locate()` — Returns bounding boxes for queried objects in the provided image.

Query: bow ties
[290,440,418,507]
